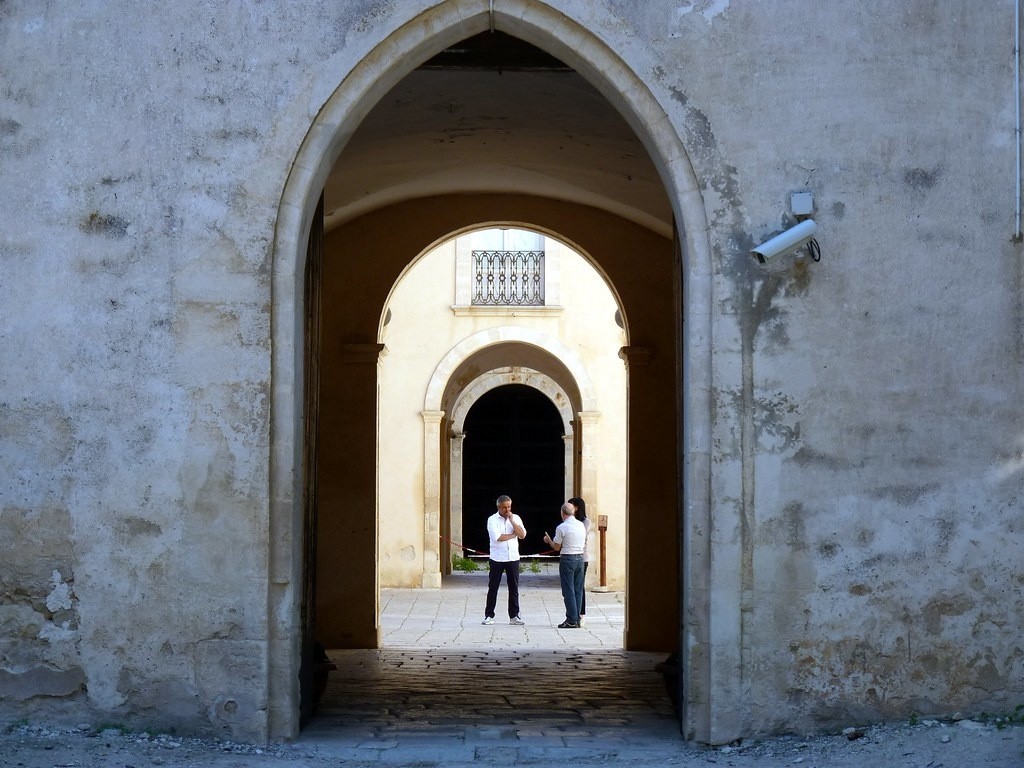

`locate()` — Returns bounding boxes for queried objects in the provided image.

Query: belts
[561,554,583,557]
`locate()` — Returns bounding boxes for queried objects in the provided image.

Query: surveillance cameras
[749,219,817,268]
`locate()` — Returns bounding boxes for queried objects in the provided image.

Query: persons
[482,495,527,625]
[569,496,591,616]
[544,503,586,628]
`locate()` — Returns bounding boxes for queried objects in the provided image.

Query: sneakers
[509,616,525,625]
[482,617,494,624]
[558,620,580,628]
